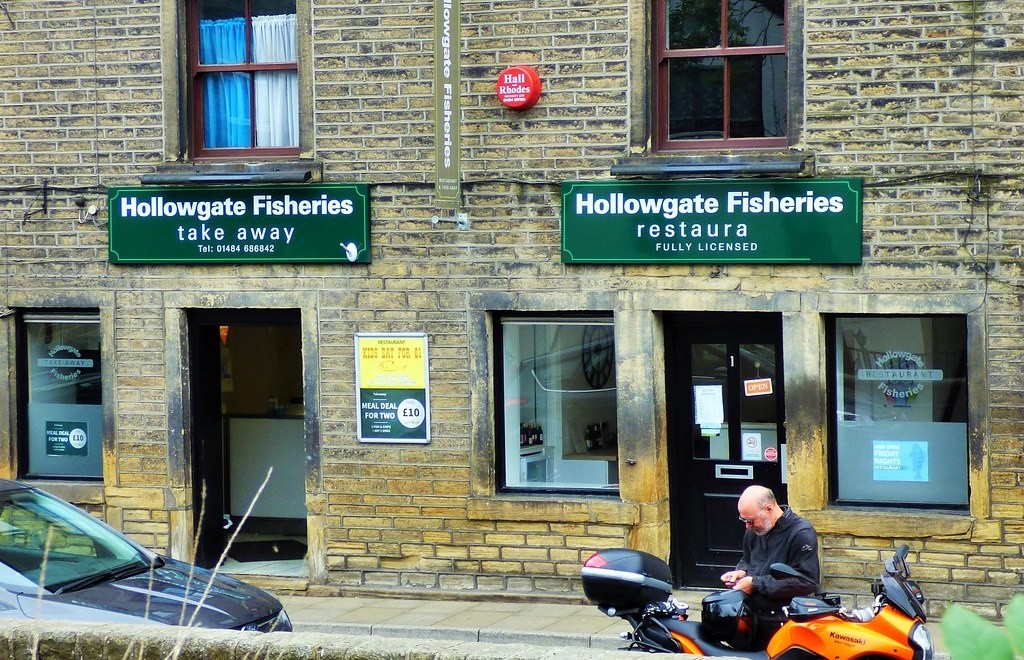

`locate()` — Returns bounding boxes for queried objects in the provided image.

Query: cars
[0,475,294,634]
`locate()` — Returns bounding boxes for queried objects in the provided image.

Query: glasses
[739,508,765,524]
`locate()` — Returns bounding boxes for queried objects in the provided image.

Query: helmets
[701,589,757,651]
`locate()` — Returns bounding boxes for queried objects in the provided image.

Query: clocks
[582,325,612,389]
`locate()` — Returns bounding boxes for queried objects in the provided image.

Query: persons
[720,484,820,639]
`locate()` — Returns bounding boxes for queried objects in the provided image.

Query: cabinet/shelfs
[520,445,555,483]
[222,413,306,535]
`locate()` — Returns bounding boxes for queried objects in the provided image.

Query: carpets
[226,540,308,562]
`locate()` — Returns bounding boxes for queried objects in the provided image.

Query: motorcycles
[581,541,934,660]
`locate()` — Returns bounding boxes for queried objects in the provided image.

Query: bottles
[520,421,544,446]
[585,425,603,451]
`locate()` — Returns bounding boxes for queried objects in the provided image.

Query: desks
[562,439,619,484]
